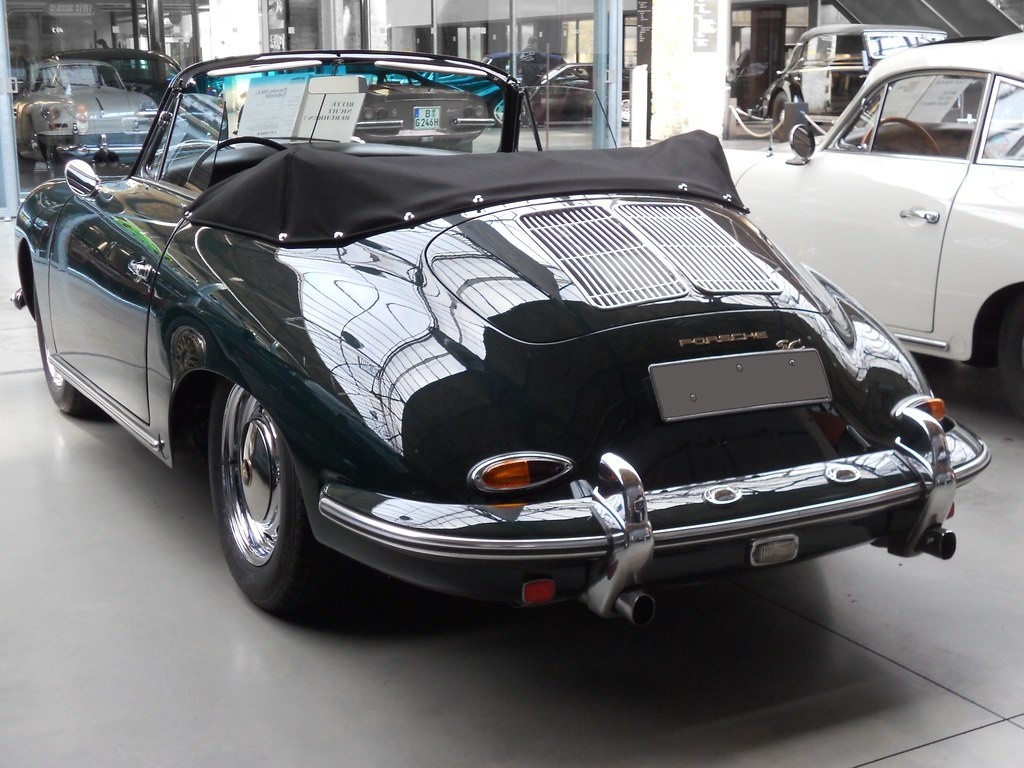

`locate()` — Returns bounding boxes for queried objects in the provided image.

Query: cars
[763,21,953,141]
[482,51,565,75]
[516,61,633,131]
[56,48,182,98]
[724,36,1024,423]
[11,60,162,166]
[193,51,504,126]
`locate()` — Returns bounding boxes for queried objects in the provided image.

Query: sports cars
[12,52,987,629]
